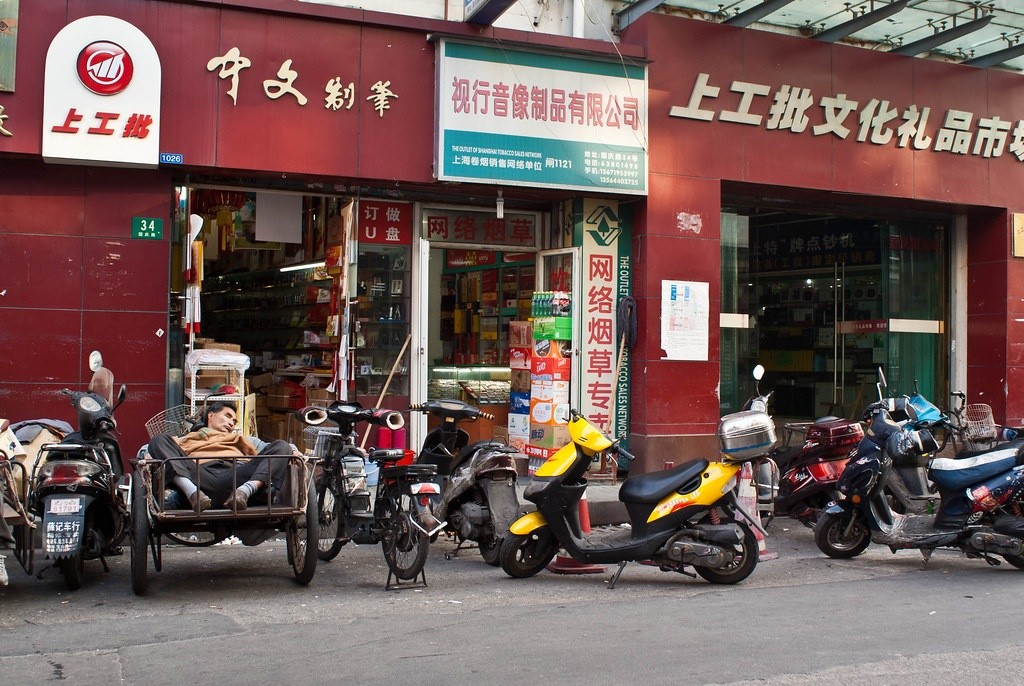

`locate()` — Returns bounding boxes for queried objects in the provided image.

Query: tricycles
[130,391,324,594]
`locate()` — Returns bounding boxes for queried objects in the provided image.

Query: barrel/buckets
[382,449,415,486]
[363,457,380,487]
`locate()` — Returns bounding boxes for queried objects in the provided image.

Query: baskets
[960,404,998,452]
[145,404,198,440]
[301,425,359,457]
[741,395,768,415]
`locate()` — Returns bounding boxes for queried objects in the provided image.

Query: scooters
[0,417,75,542]
[292,400,448,591]
[33,349,132,590]
[814,365,1024,570]
[500,407,778,589]
[406,400,522,568]
[741,363,864,531]
[897,378,960,457]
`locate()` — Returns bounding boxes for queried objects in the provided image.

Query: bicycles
[930,391,999,457]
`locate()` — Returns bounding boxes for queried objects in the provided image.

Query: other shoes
[0,555,8,585]
[189,490,211,512]
[224,488,247,510]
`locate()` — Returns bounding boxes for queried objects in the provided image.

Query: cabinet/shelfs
[184,353,246,437]
[444,260,536,366]
[738,266,887,383]
[202,264,408,378]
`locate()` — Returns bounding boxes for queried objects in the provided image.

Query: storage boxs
[187,338,335,453]
[751,281,882,418]
[442,248,571,477]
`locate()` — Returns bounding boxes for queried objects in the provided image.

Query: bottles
[527,457,547,480]
[531,291,572,318]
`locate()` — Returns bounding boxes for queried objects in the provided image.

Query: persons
[146,402,304,512]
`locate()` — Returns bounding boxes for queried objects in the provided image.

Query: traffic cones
[547,489,608,573]
[734,459,779,562]
